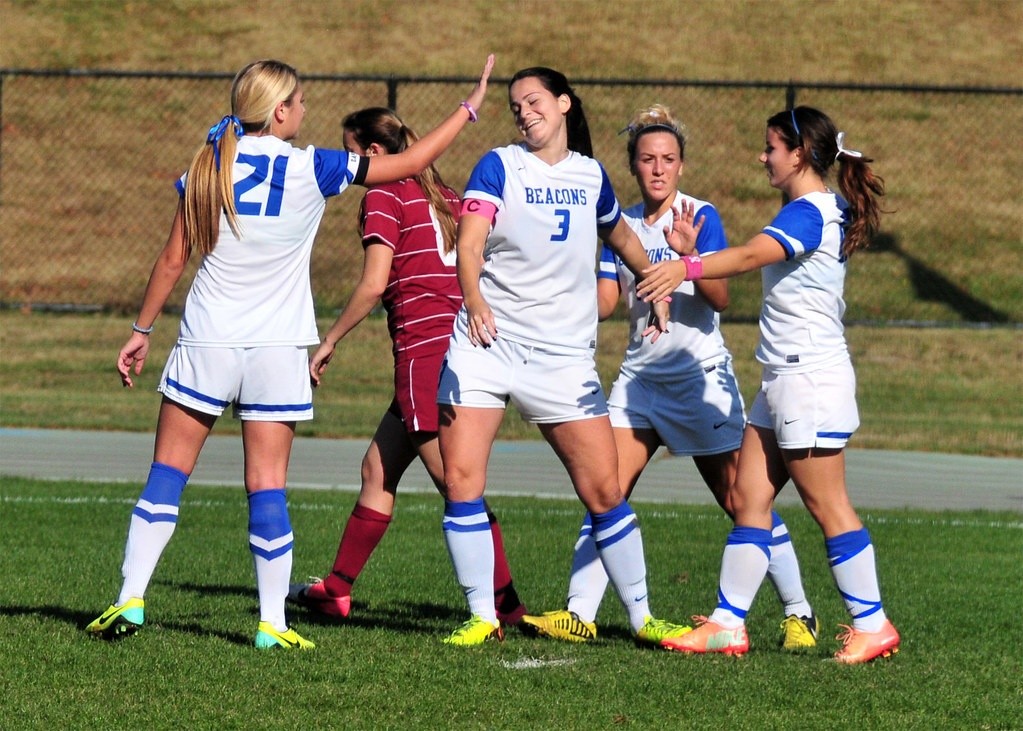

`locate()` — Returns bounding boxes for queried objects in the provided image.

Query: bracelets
[461,100,479,125]
[662,297,673,304]
[131,321,155,336]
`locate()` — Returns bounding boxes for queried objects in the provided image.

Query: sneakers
[781,612,820,648]
[287,583,351,617]
[661,620,749,657]
[494,604,528,624]
[254,622,316,649]
[86,599,145,636]
[629,616,692,647]
[441,619,504,647]
[518,609,597,643]
[834,620,900,663]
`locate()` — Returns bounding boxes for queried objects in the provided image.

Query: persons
[286,107,533,627]
[435,65,701,650]
[84,50,499,648]
[512,100,822,652]
[633,99,905,667]
